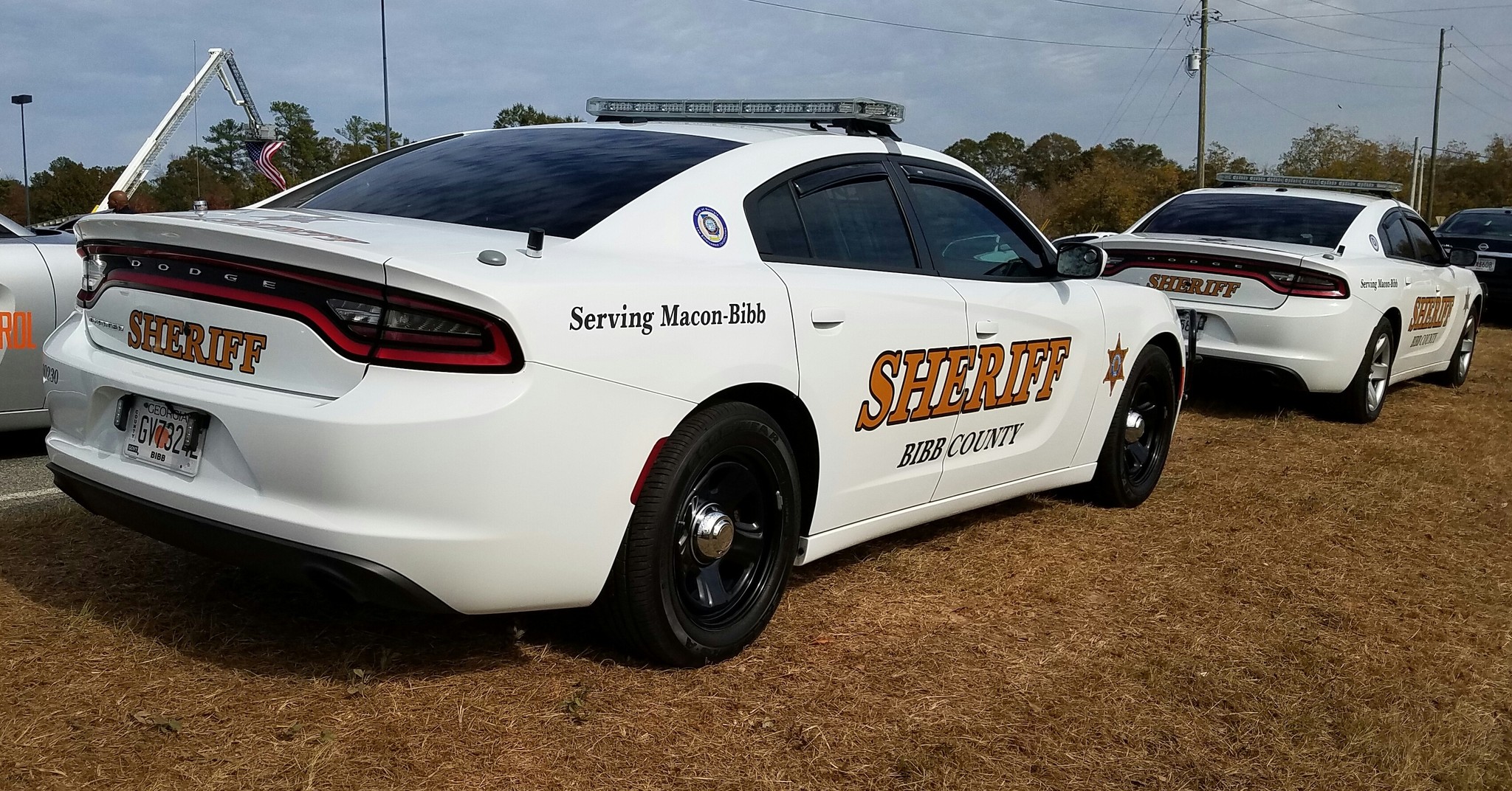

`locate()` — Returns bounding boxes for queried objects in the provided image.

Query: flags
[244,141,287,191]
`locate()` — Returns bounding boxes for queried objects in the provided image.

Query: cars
[43,88,1178,665]
[1081,175,1484,423]
[1436,202,1511,324]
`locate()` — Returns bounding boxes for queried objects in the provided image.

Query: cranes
[97,45,276,214]
